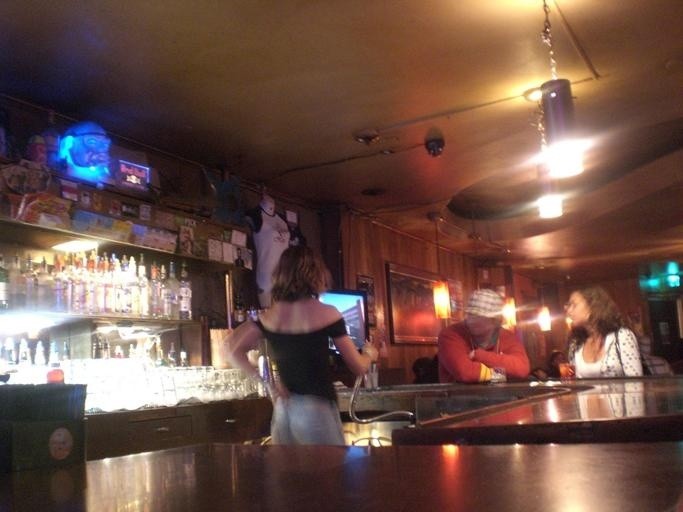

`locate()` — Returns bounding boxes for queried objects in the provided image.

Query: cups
[555,357,570,380]
[360,346,379,391]
[490,367,507,385]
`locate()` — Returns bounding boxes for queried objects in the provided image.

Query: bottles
[231,294,266,327]
[379,340,392,391]
[44,368,64,387]
[0,249,192,366]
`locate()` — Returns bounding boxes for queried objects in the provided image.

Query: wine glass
[79,364,271,413]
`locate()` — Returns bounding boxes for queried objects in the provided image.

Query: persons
[246,193,299,307]
[228,245,378,446]
[436,288,531,382]
[564,284,643,380]
[413,350,573,384]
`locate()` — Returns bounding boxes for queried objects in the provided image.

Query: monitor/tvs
[322,291,369,353]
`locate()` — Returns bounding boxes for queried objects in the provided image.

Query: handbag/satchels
[622,358,651,376]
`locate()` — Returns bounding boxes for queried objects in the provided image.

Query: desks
[1,441,683,512]
[337,377,683,444]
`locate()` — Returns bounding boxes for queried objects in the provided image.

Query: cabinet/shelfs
[0,219,239,365]
[84,399,274,460]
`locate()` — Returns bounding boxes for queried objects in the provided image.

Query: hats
[464,289,504,318]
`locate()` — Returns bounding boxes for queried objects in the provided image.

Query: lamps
[536,1,579,219]
